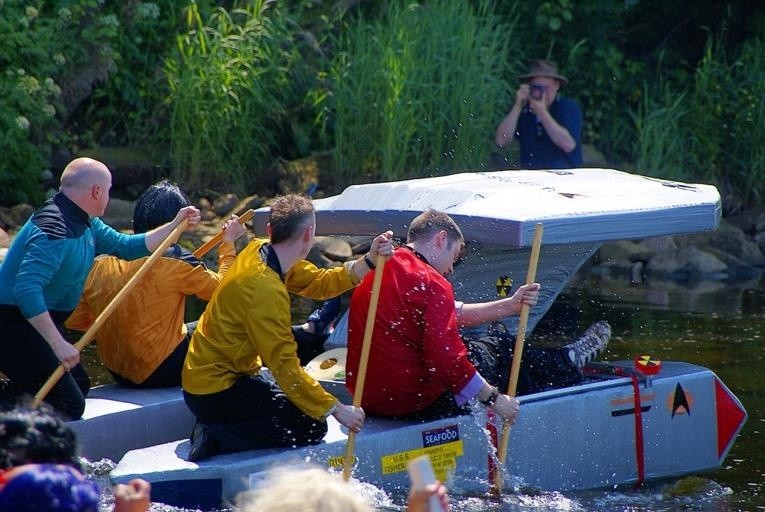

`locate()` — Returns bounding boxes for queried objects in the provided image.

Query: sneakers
[488,319,509,336]
[562,320,612,370]
[307,296,342,336]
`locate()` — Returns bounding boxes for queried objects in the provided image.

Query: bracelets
[363,254,378,270]
[478,386,501,406]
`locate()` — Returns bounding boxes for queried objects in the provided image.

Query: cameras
[528,83,544,100]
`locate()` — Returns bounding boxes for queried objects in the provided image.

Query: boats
[28,165,749,512]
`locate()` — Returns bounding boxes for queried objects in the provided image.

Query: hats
[518,60,568,91]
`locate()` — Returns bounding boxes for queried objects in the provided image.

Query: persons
[495,59,586,168]
[0,402,84,473]
[53,179,343,390]
[226,455,454,512]
[0,461,154,512]
[0,155,200,420]
[339,205,614,425]
[177,192,399,463]
